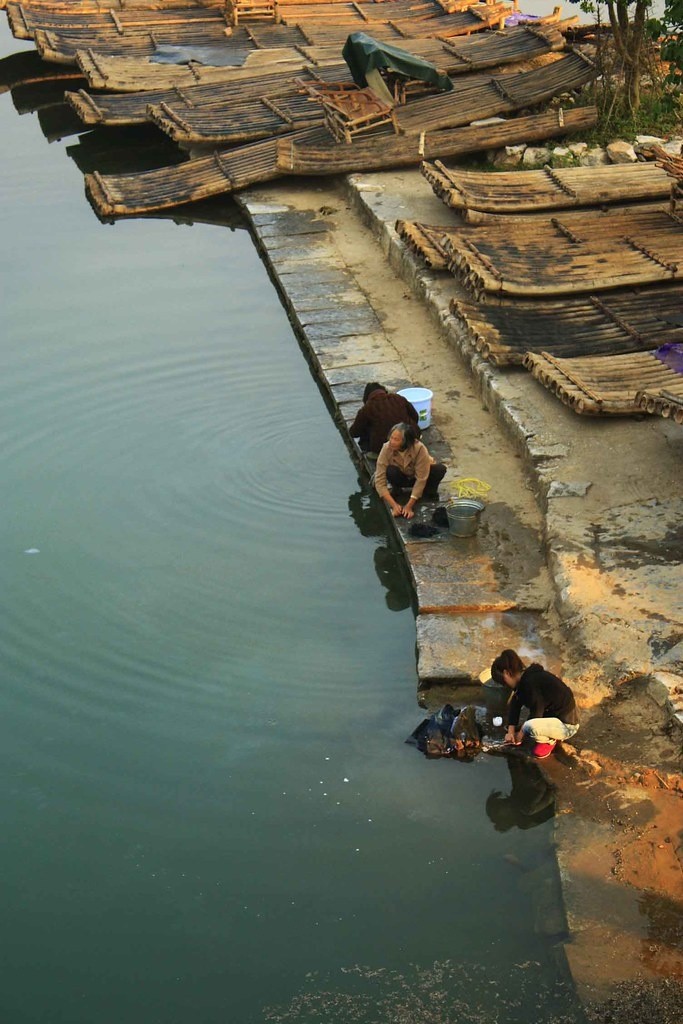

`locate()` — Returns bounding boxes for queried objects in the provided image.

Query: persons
[349,382,421,460]
[367,422,447,519]
[492,650,580,759]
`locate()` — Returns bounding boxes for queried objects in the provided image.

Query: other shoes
[366,450,379,460]
[422,491,440,499]
[388,488,404,496]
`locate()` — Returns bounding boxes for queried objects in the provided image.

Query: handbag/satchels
[403,703,482,758]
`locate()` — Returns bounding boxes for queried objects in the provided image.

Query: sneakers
[505,718,527,732]
[532,738,557,759]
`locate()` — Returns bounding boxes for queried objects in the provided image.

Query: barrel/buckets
[478,667,516,712]
[444,499,485,538]
[396,387,434,429]
